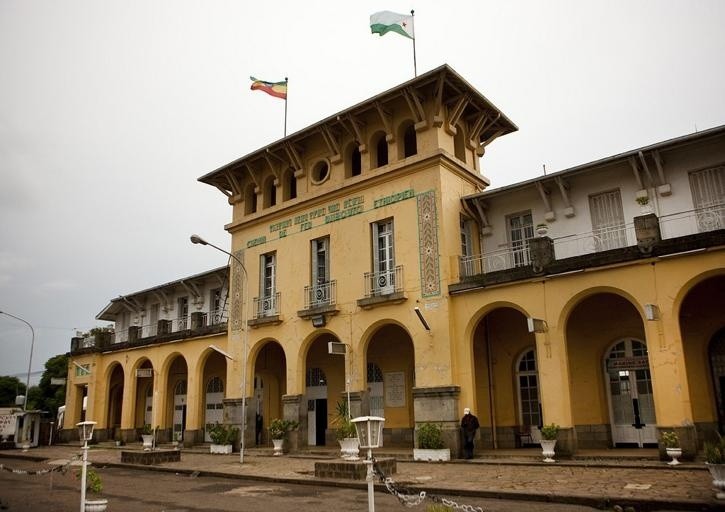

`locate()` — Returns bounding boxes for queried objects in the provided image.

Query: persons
[256,411,263,445]
[460,408,480,459]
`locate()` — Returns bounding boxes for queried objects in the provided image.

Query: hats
[464,408,471,415]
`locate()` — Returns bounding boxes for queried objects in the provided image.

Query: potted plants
[210,426,239,454]
[268,420,298,455]
[413,422,451,462]
[664,432,684,465]
[330,401,360,461]
[138,424,159,447]
[114,432,124,446]
[539,423,560,462]
[536,223,548,237]
[76,467,108,512]
[704,442,725,499]
[635,196,651,214]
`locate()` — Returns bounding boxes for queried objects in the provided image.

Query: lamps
[413,306,434,337]
[312,315,326,327]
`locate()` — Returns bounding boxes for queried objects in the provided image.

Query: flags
[369,11,413,40]
[250,76,287,98]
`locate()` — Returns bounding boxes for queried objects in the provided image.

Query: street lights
[188,232,251,466]
[48,421,55,446]
[0,310,35,411]
[73,421,98,512]
[347,414,388,512]
